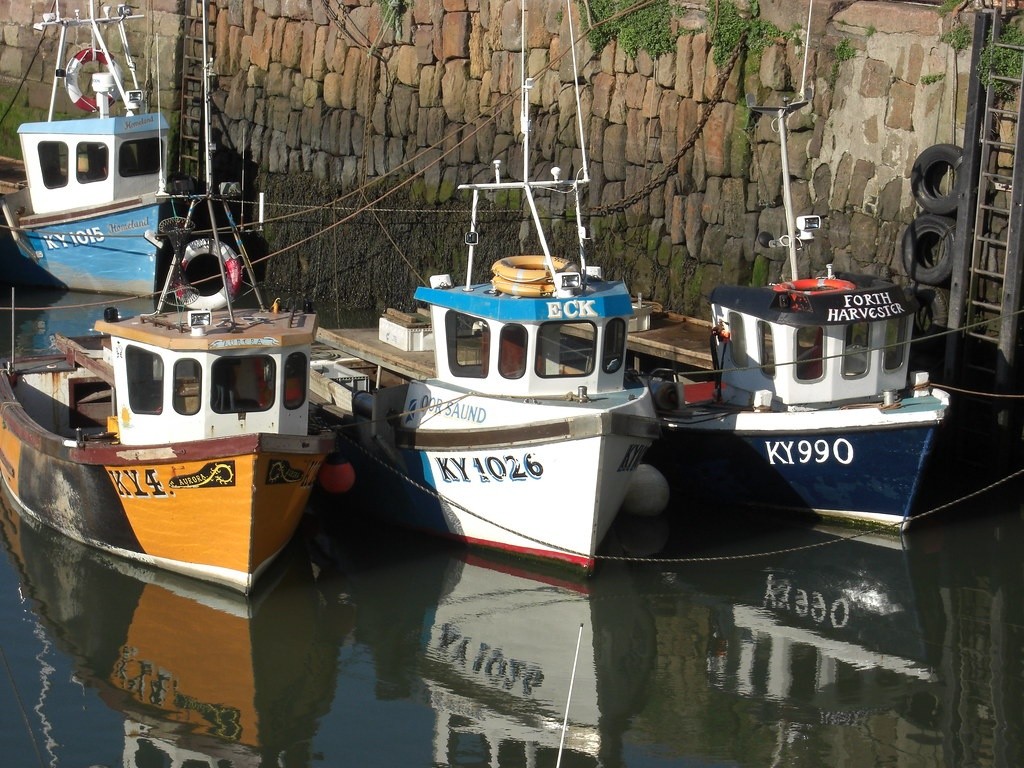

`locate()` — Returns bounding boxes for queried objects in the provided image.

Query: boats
[364,533,659,768]
[309,0,664,580]
[0,0,195,299]
[560,2,952,534]
[1,495,354,767]
[675,520,947,768]
[0,0,338,599]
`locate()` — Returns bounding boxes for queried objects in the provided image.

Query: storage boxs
[565,314,650,333]
[379,317,435,352]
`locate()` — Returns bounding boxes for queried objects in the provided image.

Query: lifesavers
[171,237,242,312]
[65,49,125,113]
[900,216,956,288]
[770,279,855,303]
[910,144,972,215]
[492,255,576,297]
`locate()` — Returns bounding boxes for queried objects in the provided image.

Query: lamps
[555,272,582,298]
[797,215,821,240]
[126,90,143,108]
[187,310,212,337]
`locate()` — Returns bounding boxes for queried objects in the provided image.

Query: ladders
[961,6,1024,431]
